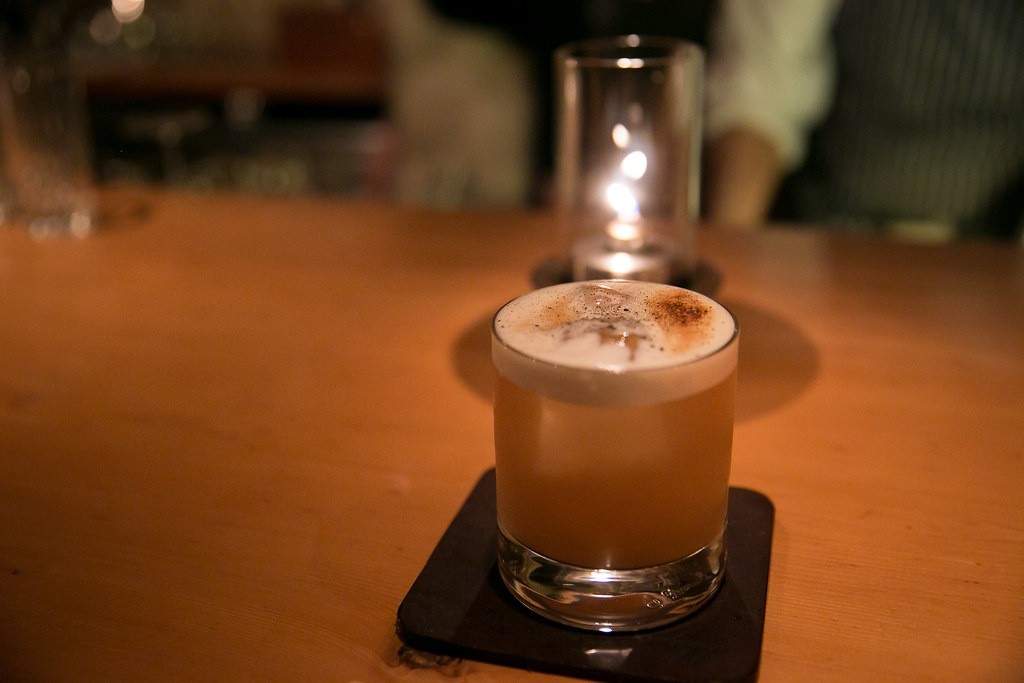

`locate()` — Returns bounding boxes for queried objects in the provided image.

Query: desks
[2,173,1023,682]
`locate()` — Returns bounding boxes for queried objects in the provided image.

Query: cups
[0,0,163,239]
[491,279,740,632]
[542,38,703,286]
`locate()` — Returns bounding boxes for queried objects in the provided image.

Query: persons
[375,0,836,222]
